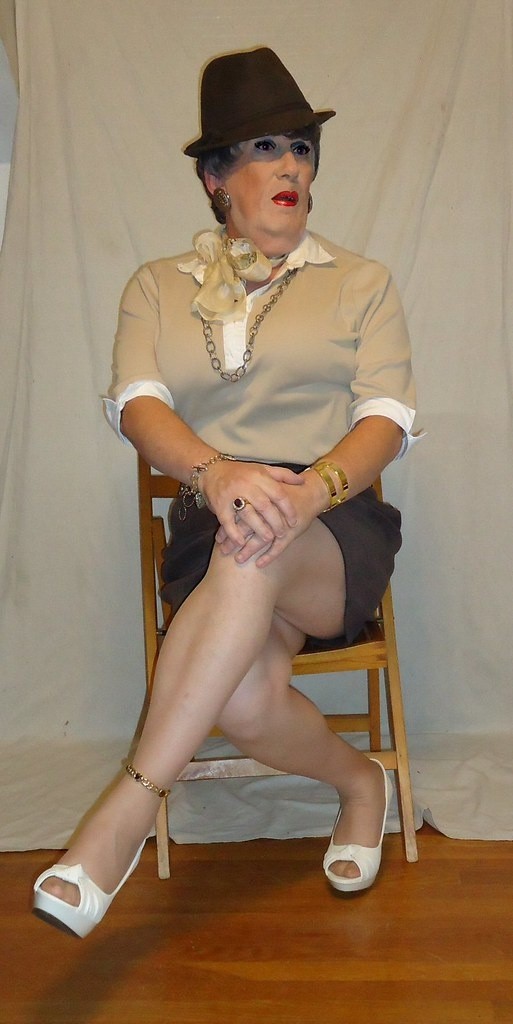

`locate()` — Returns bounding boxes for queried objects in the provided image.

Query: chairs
[137,450,419,880]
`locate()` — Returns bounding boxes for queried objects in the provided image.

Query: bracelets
[303,461,349,512]
[190,452,236,508]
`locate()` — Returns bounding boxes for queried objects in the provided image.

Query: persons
[31,48,427,940]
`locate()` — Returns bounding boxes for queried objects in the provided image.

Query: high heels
[322,758,393,891]
[32,836,150,939]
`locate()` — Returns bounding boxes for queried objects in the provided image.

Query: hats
[184,47,337,157]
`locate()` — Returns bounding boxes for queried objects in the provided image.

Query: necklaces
[201,268,300,381]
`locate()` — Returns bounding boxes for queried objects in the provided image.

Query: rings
[232,497,251,516]
